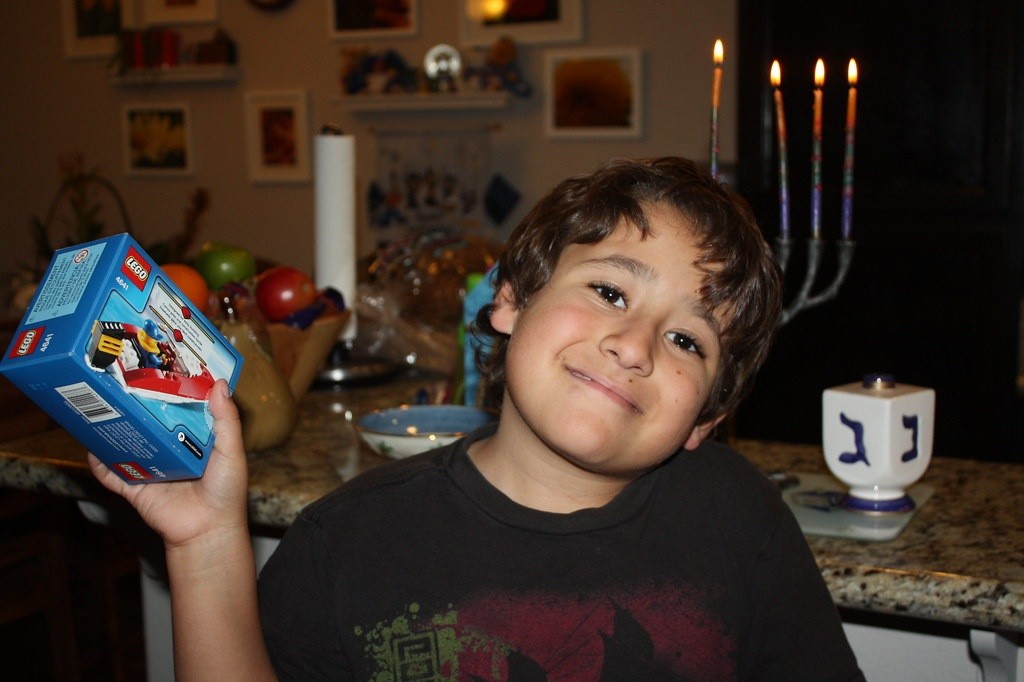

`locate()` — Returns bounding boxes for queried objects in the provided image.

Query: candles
[769,59,789,139]
[814,58,825,140]
[847,58,858,132]
[712,40,725,106]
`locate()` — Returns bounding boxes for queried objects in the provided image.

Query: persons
[86,156,866,682]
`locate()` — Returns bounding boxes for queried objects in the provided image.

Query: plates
[353,404,489,460]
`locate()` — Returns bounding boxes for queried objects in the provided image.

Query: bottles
[451,273,487,404]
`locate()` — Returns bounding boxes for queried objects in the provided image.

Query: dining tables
[0,373,1024,682]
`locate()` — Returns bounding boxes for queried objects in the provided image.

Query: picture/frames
[0,232,242,484]
[65,1,644,184]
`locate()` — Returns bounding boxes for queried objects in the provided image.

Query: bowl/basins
[215,310,351,407]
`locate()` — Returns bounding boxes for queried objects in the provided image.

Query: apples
[156,241,314,327]
